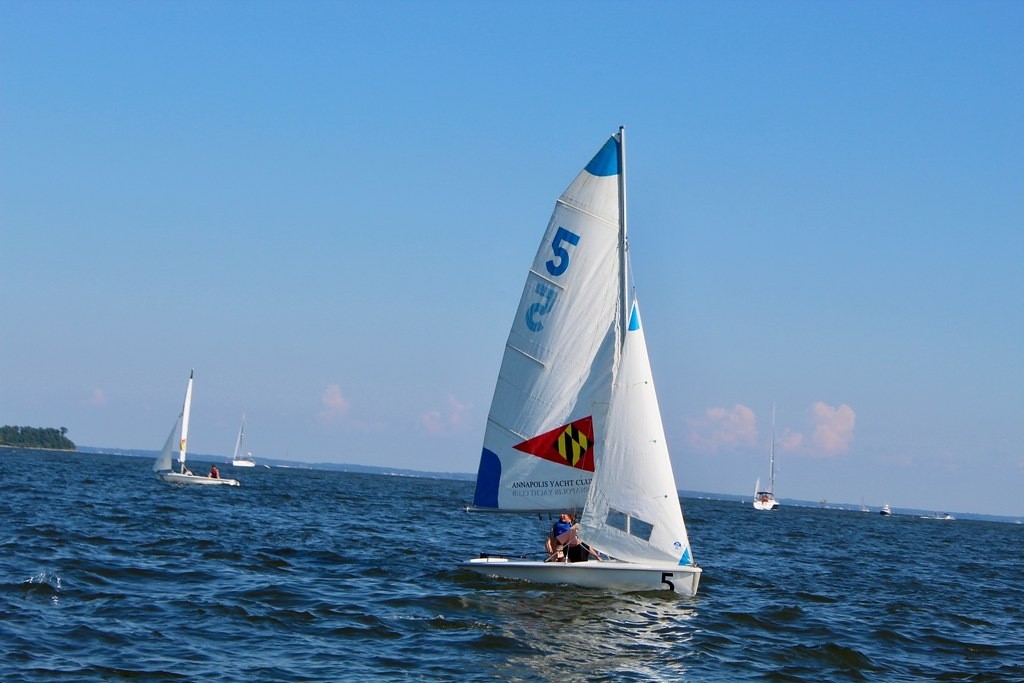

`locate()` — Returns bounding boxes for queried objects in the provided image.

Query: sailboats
[752,406,780,514]
[456,127,703,600]
[231,414,257,468]
[150,367,242,487]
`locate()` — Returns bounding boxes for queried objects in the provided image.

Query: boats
[880,504,892,517]
[943,513,953,521]
[861,506,870,513]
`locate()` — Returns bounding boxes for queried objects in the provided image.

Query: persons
[554,523,603,562]
[546,512,582,561]
[208,464,219,478]
[762,494,768,504]
